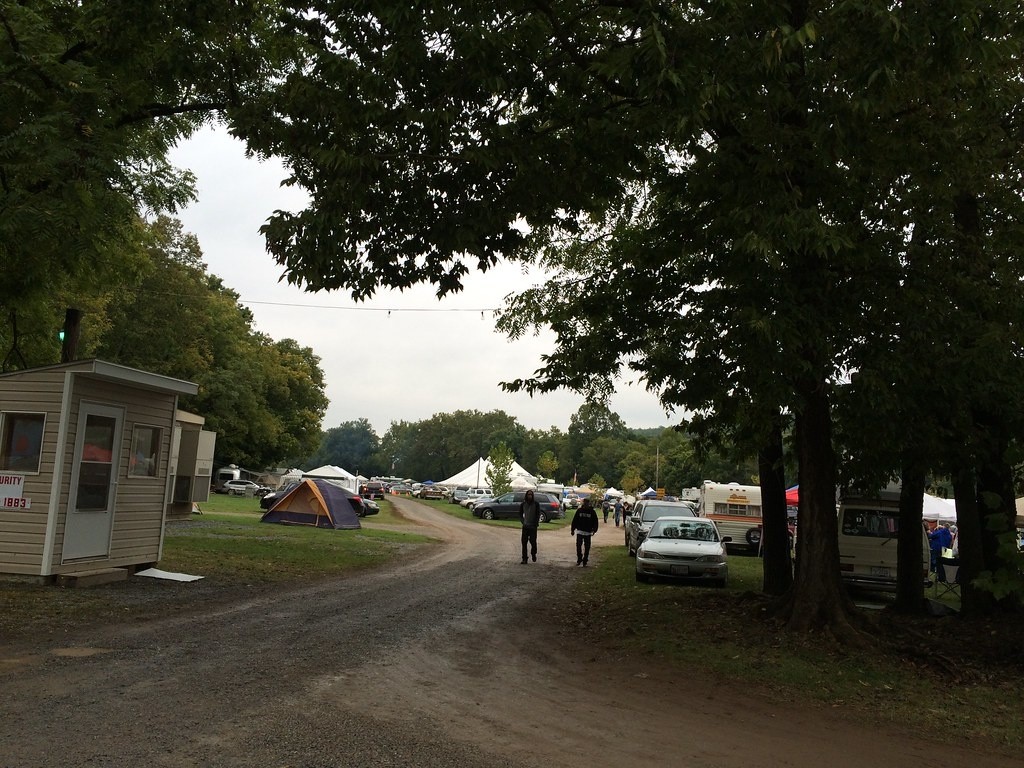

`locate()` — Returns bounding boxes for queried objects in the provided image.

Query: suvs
[624,499,698,558]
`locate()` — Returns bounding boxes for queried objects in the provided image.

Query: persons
[1016,528,1024,552]
[601,499,610,523]
[570,499,598,568]
[622,501,630,527]
[613,498,622,527]
[922,519,959,585]
[518,490,540,564]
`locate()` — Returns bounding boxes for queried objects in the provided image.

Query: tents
[433,457,514,490]
[603,486,625,499]
[275,464,367,495]
[639,487,668,498]
[423,480,434,484]
[258,479,362,530]
[484,451,555,484]
[402,479,417,484]
[1014,496,1024,526]
[785,483,800,507]
[503,475,536,491]
[921,491,960,524]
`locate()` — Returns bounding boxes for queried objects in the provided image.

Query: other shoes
[583,562,588,567]
[521,559,527,564]
[577,559,582,566]
[532,554,536,562]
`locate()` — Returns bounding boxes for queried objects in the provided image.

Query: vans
[788,490,936,588]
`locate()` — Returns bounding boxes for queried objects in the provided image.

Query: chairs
[695,528,709,538]
[934,555,963,601]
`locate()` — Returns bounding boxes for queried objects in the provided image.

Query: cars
[259,479,582,523]
[633,516,733,589]
[220,480,270,497]
